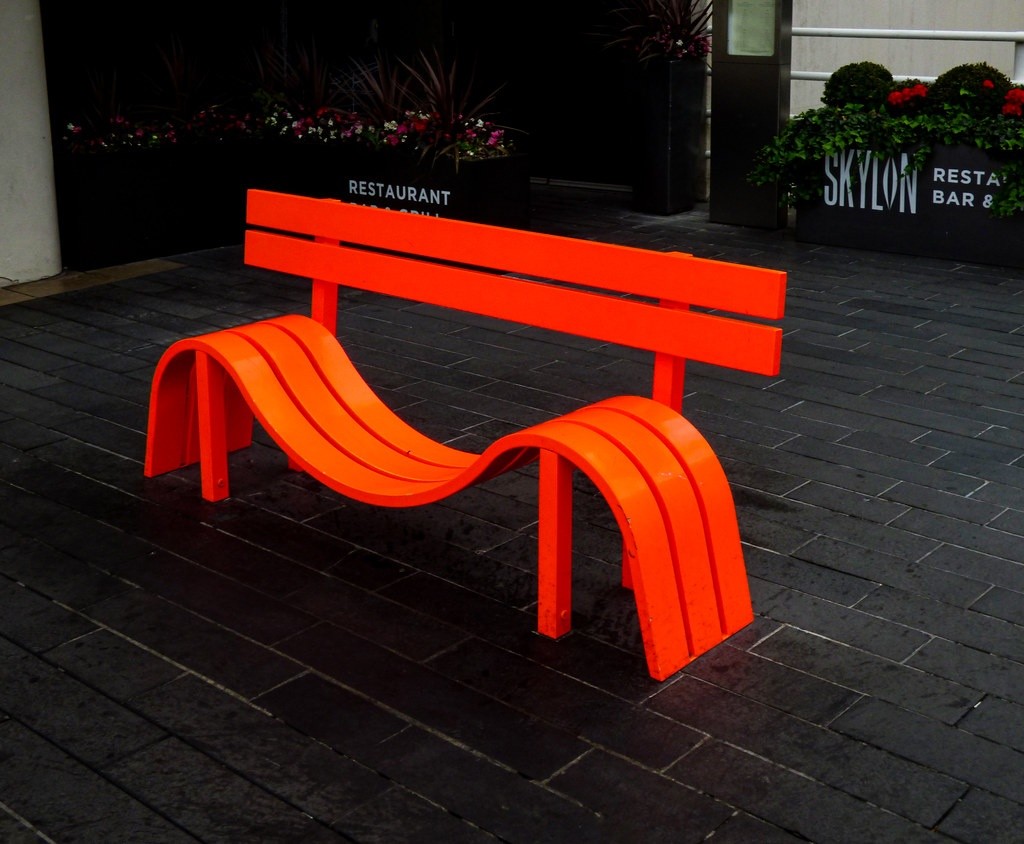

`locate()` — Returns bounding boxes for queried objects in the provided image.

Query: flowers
[584,0,713,71]
[64,36,530,174]
[745,62,1024,219]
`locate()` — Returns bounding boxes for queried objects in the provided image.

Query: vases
[796,144,1024,269]
[65,151,530,266]
[629,59,706,214]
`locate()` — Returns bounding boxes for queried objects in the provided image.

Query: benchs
[145,188,787,681]
